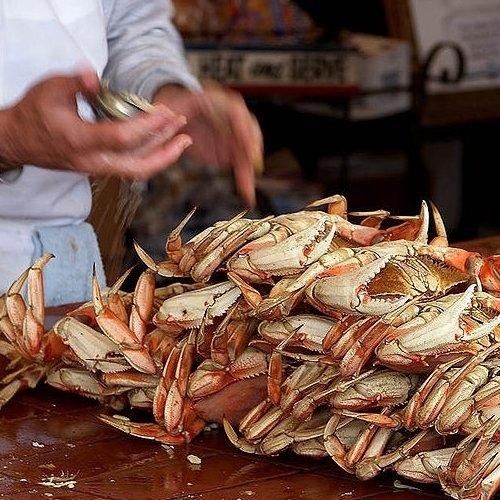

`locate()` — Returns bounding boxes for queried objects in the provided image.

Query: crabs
[1,187,500,499]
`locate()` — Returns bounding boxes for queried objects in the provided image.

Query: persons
[1,1,267,314]
